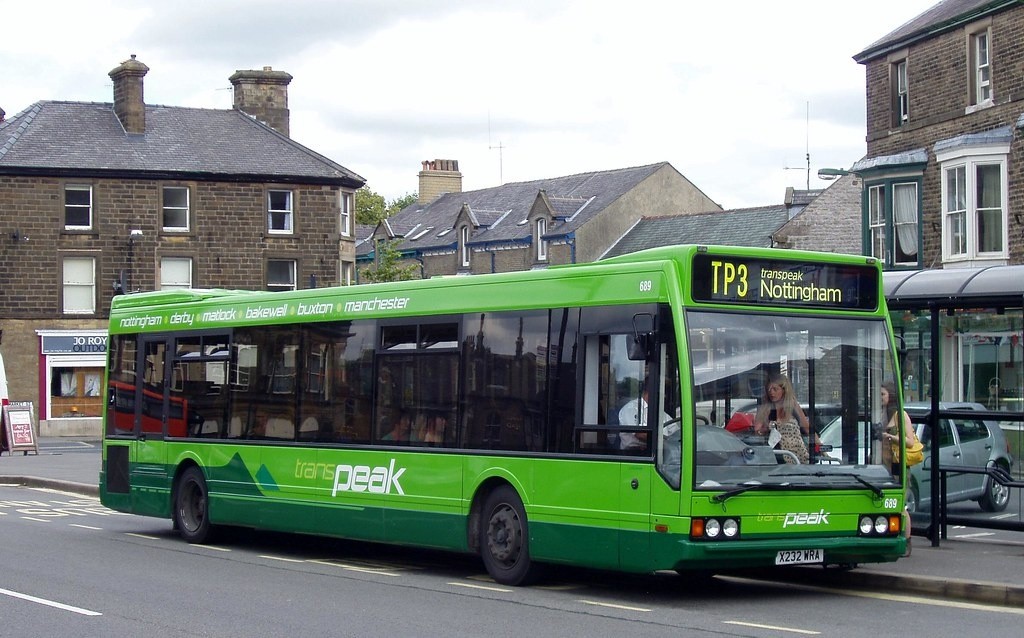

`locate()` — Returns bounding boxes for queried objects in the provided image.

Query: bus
[96,244,913,585]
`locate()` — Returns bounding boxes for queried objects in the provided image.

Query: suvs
[672,398,1013,518]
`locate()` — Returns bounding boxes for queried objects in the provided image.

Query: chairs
[190,408,414,446]
[607,397,631,443]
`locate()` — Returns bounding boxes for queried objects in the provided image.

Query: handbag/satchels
[890,410,924,466]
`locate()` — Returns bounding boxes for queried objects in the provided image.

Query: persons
[871,378,917,556]
[615,374,683,459]
[183,335,601,458]
[753,374,828,464]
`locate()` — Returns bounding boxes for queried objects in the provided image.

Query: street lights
[818,167,868,257]
